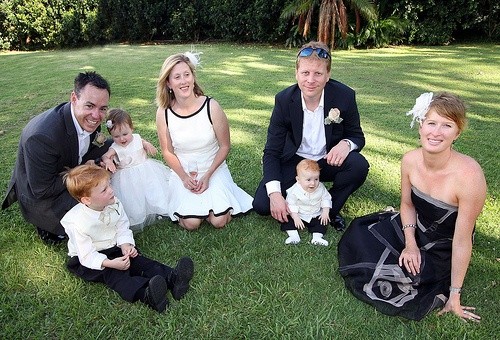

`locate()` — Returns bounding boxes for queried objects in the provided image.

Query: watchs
[342,140,352,150]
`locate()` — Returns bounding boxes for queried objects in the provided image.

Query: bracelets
[401,224,417,231]
[450,286,461,295]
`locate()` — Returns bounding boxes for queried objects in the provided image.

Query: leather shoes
[36,225,64,245]
[328,209,346,231]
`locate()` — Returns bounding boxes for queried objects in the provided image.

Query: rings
[463,310,468,314]
[407,260,412,263]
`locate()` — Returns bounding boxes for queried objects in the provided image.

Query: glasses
[296,47,331,61]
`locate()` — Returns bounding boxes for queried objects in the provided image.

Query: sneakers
[141,257,195,313]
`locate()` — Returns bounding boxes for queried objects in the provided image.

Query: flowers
[105,119,114,129]
[92,131,108,148]
[325,108,343,125]
[407,92,434,128]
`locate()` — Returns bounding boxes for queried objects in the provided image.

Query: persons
[60,165,194,313]
[337,91,487,324]
[2,71,117,248]
[155,51,254,230]
[100,109,158,226]
[279,159,332,247]
[252,42,370,230]
